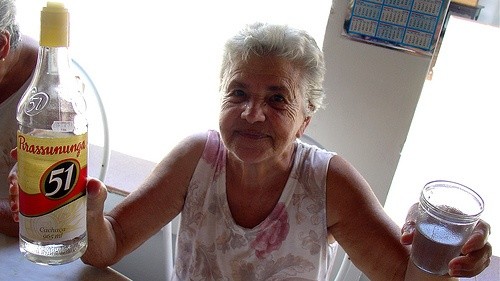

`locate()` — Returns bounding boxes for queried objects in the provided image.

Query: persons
[0,1,109,240]
[7,24,492,281]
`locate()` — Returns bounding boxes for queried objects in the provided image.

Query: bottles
[16,2,89,265]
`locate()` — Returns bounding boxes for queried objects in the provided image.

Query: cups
[409,180,484,276]
